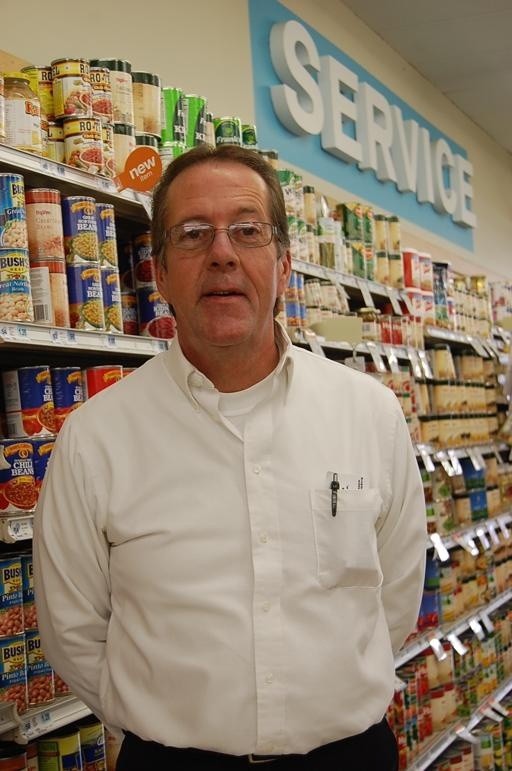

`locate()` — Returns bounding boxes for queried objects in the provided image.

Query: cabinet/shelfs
[0,143,512,770]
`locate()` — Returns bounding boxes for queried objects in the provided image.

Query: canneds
[385,630,512,771]
[0,362,139,771]
[0,56,436,349]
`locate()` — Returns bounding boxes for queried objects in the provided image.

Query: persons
[30,145,431,771]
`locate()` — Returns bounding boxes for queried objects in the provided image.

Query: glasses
[157,223,283,250]
